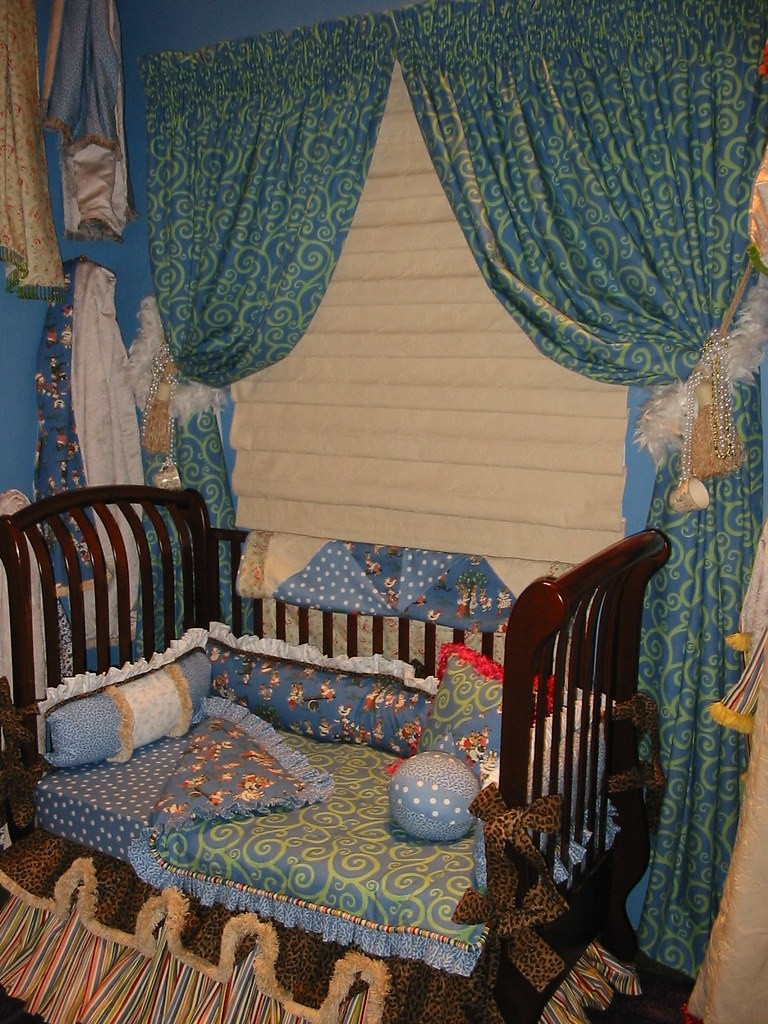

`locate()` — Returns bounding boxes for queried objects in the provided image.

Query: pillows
[38,629,211,769]
[385,643,564,791]
[206,621,441,760]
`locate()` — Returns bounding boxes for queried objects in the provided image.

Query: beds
[0,484,671,1024]
